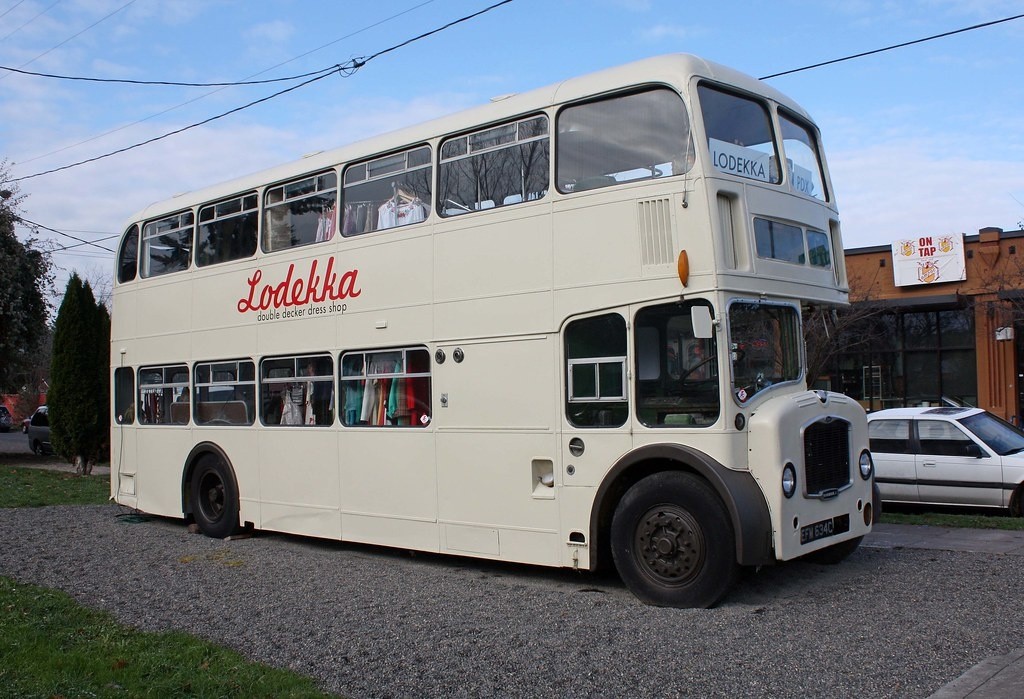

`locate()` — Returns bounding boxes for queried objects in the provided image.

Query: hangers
[385,181,421,208]
[325,200,383,212]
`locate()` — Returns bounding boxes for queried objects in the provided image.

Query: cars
[28,405,59,457]
[867,408,1024,518]
[20,418,32,433]
[0,405,13,433]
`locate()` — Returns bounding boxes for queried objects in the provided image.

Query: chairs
[568,335,620,425]
[573,175,617,192]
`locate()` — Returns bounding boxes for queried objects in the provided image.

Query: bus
[109,54,875,611]
